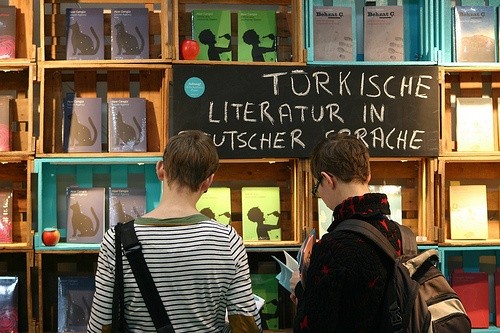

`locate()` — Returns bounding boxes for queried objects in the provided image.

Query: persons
[289,134,420,333]
[86,130,265,333]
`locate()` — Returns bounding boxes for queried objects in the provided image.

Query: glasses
[177,131,211,138]
[311,171,333,198]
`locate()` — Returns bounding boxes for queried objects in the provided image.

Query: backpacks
[331,219,471,333]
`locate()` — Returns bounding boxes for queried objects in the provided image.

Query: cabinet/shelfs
[0,0,500,333]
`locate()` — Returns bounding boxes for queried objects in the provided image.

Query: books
[66,187,146,244]
[0,98,12,152]
[0,7,16,59]
[191,10,277,62]
[0,181,13,244]
[271,228,316,294]
[450,185,488,239]
[319,185,402,240]
[58,276,96,333]
[66,8,149,60]
[195,187,281,241]
[452,6,500,62]
[313,5,403,62]
[251,274,278,330]
[0,276,18,333]
[225,294,265,322]
[61,97,147,152]
[451,272,500,328]
[455,97,500,152]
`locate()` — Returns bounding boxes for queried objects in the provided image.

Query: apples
[179,39,200,59]
[42,230,60,246]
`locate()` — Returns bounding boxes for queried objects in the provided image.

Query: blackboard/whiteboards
[170,62,439,158]
